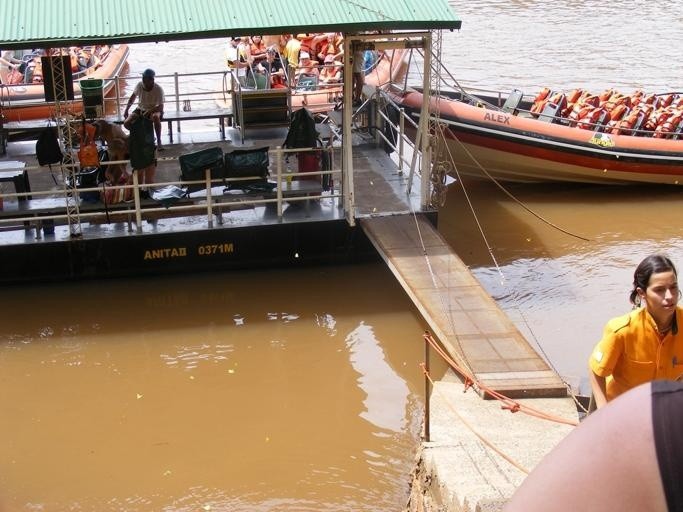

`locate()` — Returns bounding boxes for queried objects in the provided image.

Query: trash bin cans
[79,79,105,118]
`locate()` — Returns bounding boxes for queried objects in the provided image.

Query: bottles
[286,166,293,185]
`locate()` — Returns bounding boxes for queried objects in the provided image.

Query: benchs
[4,107,234,155]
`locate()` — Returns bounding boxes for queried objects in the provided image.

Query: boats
[0,43,133,125]
[238,30,411,120]
[384,76,683,193]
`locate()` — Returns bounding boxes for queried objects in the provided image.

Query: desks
[0,160,31,226]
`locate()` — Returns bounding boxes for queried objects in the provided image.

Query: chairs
[179,146,278,193]
[502,87,683,140]
[314,95,370,148]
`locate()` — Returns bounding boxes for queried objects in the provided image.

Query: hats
[299,52,310,60]
[324,54,335,63]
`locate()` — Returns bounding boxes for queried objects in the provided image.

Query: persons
[123,68,164,151]
[96,119,129,183]
[228,30,391,104]
[588,255,683,408]
[0,45,110,88]
